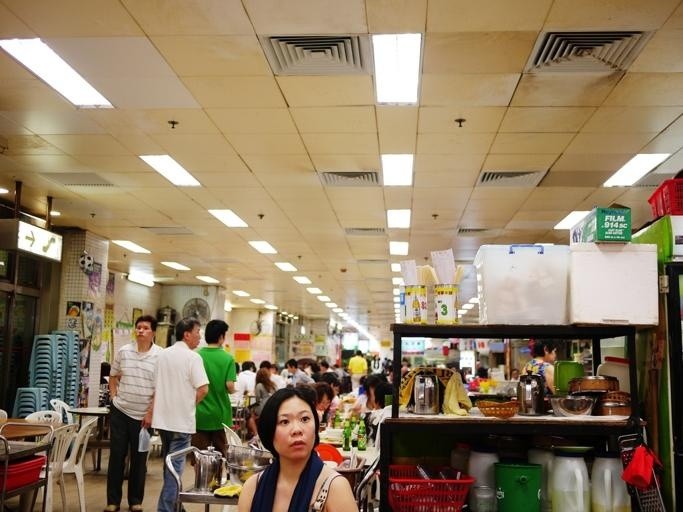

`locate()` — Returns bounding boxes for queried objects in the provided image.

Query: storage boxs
[470,242,569,327]
[568,200,633,244]
[568,243,661,327]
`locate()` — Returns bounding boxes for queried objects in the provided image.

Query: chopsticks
[438,471,452,492]
[415,463,434,487]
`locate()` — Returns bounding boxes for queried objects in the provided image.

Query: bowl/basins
[227,443,271,467]
[227,464,266,488]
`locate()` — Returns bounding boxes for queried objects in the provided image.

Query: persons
[102,315,165,512]
[151,317,210,512]
[234,386,360,512]
[97,361,111,407]
[189,319,238,467]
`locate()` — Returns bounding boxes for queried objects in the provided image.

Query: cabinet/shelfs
[380,322,645,511]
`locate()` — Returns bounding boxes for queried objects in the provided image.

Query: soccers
[79,254,93,270]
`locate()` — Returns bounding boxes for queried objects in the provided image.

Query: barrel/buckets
[494,462,544,512]
[591,456,632,512]
[550,456,590,512]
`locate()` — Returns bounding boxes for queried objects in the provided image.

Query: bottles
[350,412,358,448]
[357,415,367,452]
[341,417,352,452]
[332,406,342,430]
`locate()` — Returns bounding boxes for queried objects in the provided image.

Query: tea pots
[414,369,439,415]
[516,370,545,416]
[194,445,230,493]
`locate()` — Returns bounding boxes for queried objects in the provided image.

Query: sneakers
[103,505,120,512]
[129,504,144,512]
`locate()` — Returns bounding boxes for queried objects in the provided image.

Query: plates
[551,443,595,453]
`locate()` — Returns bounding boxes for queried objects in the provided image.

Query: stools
[11,329,81,419]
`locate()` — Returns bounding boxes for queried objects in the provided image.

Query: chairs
[0,399,111,511]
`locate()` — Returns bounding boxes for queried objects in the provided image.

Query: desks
[163,382,385,511]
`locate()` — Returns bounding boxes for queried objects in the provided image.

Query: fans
[179,297,210,326]
